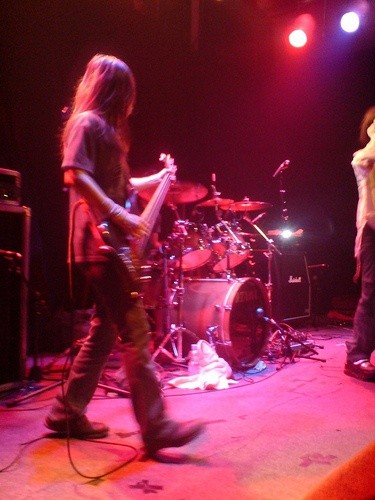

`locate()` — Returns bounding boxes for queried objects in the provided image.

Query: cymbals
[197,197,233,207]
[138,180,208,204]
[219,200,273,211]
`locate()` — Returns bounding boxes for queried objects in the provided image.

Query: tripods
[6,301,132,404]
[147,205,200,366]
[244,215,323,365]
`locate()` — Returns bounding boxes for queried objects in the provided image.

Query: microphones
[273,160,288,178]
[256,307,264,319]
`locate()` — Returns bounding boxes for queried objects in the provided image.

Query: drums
[166,220,212,273]
[139,265,176,310]
[203,221,253,272]
[163,276,270,369]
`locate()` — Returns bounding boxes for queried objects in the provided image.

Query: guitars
[97,152,177,300]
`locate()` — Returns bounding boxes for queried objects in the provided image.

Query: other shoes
[344,358,375,381]
[142,418,204,455]
[43,414,109,439]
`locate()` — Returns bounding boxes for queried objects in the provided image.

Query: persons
[343,105,375,382]
[43,54,208,455]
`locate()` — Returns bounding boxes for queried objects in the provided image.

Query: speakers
[252,249,312,322]
